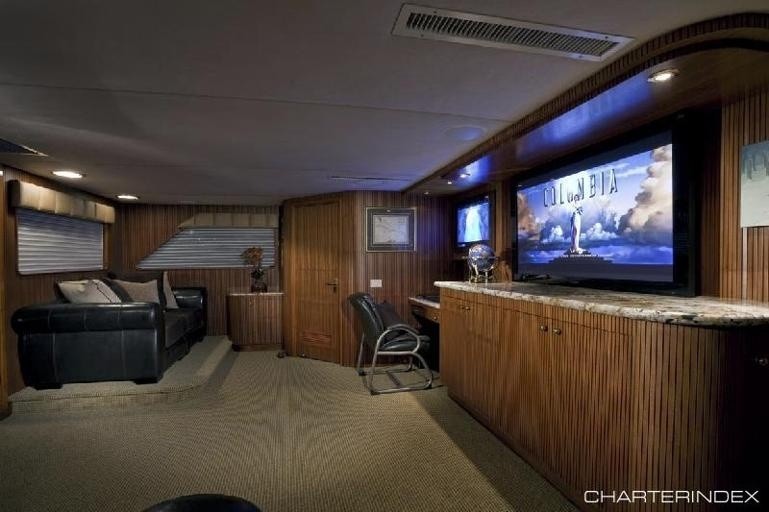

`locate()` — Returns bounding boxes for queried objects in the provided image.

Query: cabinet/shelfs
[408,294,440,369]
[437,288,631,512]
[228,292,285,352]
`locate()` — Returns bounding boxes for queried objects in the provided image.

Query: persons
[571,194,583,252]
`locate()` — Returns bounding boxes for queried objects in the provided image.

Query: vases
[250,278,268,293]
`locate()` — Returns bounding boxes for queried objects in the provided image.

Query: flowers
[239,246,265,281]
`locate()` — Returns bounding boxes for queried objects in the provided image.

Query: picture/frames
[364,206,416,254]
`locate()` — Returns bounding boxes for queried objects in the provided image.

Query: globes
[469,245,495,282]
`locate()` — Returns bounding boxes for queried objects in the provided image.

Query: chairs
[347,291,434,395]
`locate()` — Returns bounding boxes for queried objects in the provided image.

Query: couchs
[16,286,208,390]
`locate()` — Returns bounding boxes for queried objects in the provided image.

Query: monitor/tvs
[508,112,705,298]
[452,196,493,247]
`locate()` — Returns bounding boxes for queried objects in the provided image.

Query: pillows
[52,269,180,314]
